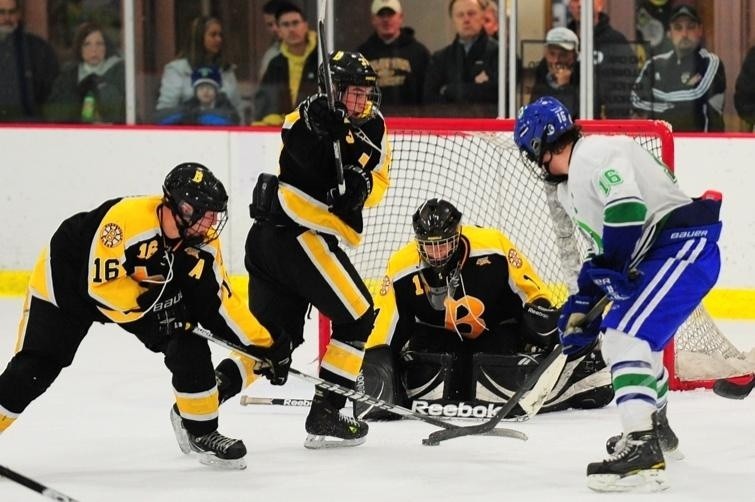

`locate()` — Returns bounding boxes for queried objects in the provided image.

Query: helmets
[514,96,581,185]
[163,162,228,250]
[319,49,382,129]
[413,198,463,273]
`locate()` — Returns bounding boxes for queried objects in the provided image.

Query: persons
[0,1,125,123]
[168,48,387,440]
[0,161,292,462]
[156,1,322,127]
[366,196,614,414]
[512,97,723,479]
[524,0,728,132]
[733,48,754,128]
[359,1,522,118]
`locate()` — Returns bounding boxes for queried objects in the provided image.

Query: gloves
[151,308,199,338]
[328,164,370,219]
[299,94,351,141]
[253,341,292,385]
[559,253,645,357]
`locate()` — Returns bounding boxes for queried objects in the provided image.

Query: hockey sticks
[428,269,644,442]
[175,323,528,442]
[714,379,754,399]
[318,1,346,196]
[241,342,571,422]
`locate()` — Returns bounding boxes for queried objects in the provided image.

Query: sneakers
[306,385,368,439]
[173,369,245,459]
[587,404,678,478]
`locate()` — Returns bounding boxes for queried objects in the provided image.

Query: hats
[191,66,222,92]
[668,5,699,25]
[371,0,402,14]
[546,27,578,50]
